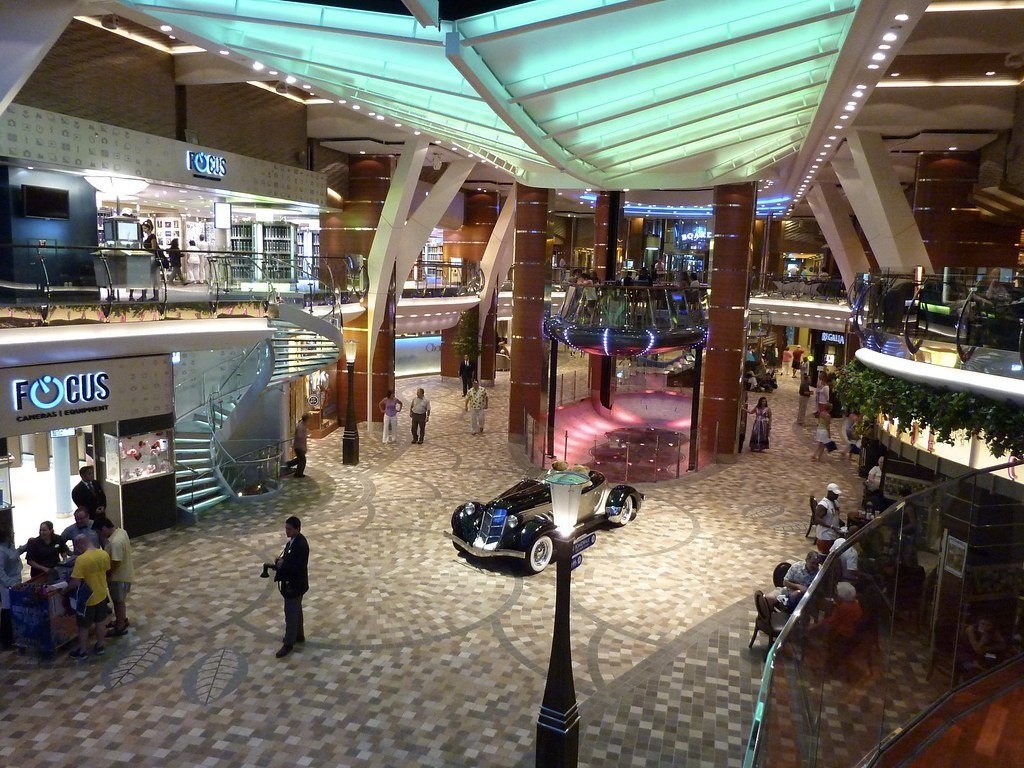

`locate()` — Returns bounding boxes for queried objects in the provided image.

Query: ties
[88,483,96,496]
[466,361,467,367]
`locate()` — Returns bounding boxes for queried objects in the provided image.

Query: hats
[796,345,800,348]
[827,483,842,494]
[807,551,821,563]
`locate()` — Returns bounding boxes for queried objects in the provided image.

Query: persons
[845,412,862,461]
[960,617,1006,683]
[98,207,208,301]
[0,508,133,659]
[274,516,309,658]
[409,388,431,444]
[465,379,488,435]
[783,457,922,667]
[459,355,474,397]
[784,265,829,281]
[560,255,700,317]
[742,396,772,452]
[71,466,107,549]
[742,343,833,424]
[811,403,833,461]
[378,391,403,443]
[285,414,309,478]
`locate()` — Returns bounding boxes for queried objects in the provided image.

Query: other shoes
[480,428,483,432]
[107,618,130,627]
[462,393,466,397]
[780,372,783,376]
[106,626,128,636]
[95,642,104,654]
[786,373,790,375]
[276,645,292,658]
[792,376,797,378]
[294,473,305,477]
[472,433,476,435]
[418,440,423,443]
[411,439,418,444]
[69,648,88,659]
[286,460,291,471]
[295,636,305,643]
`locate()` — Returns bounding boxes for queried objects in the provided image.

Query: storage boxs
[104,428,176,485]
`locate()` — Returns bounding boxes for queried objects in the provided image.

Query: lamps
[432,154,442,170]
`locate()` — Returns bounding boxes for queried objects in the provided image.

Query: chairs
[818,554,843,578]
[898,575,923,635]
[826,602,880,683]
[749,590,791,662]
[805,496,817,545]
[926,616,956,681]
[773,562,792,588]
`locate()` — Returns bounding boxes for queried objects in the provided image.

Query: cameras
[260,558,279,577]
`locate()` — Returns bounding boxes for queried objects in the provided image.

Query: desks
[916,549,941,578]
[847,512,862,530]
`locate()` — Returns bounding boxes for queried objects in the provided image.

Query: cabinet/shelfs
[231,221,320,283]
[422,245,443,277]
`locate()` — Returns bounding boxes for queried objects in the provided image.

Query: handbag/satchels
[826,437,837,453]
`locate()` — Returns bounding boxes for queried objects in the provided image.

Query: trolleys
[8,552,114,662]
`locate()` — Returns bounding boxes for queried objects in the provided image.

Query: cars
[444,462,646,574]
